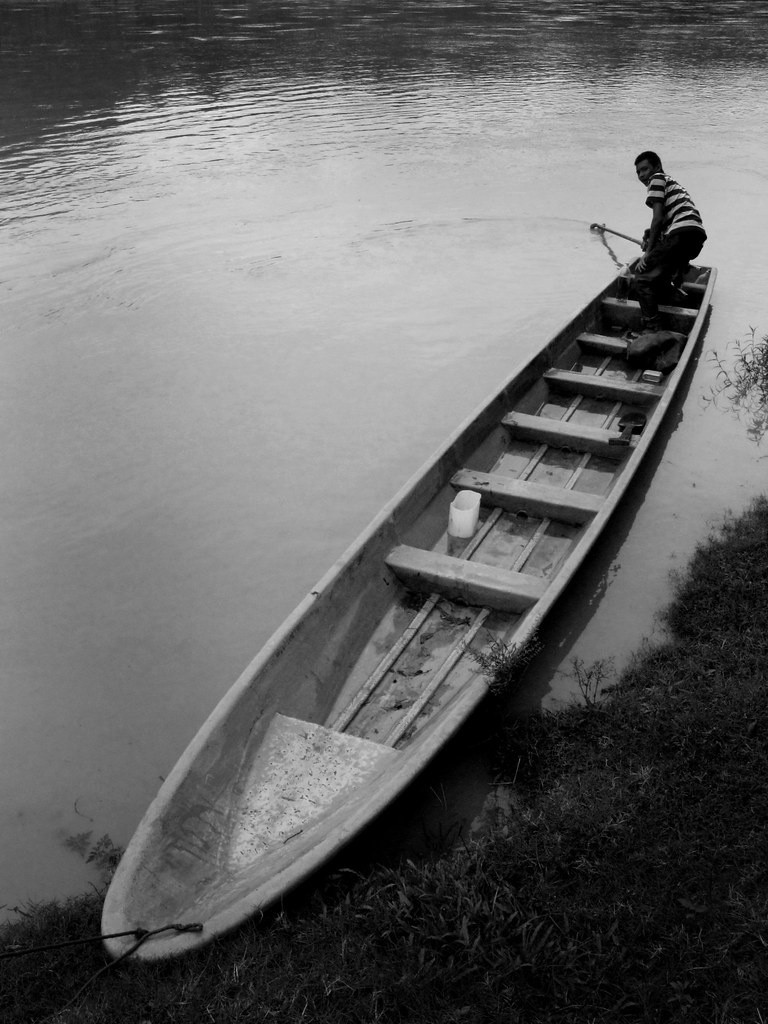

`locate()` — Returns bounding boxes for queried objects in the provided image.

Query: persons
[634,150,709,335]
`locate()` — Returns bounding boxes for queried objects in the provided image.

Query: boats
[97,256,719,973]
